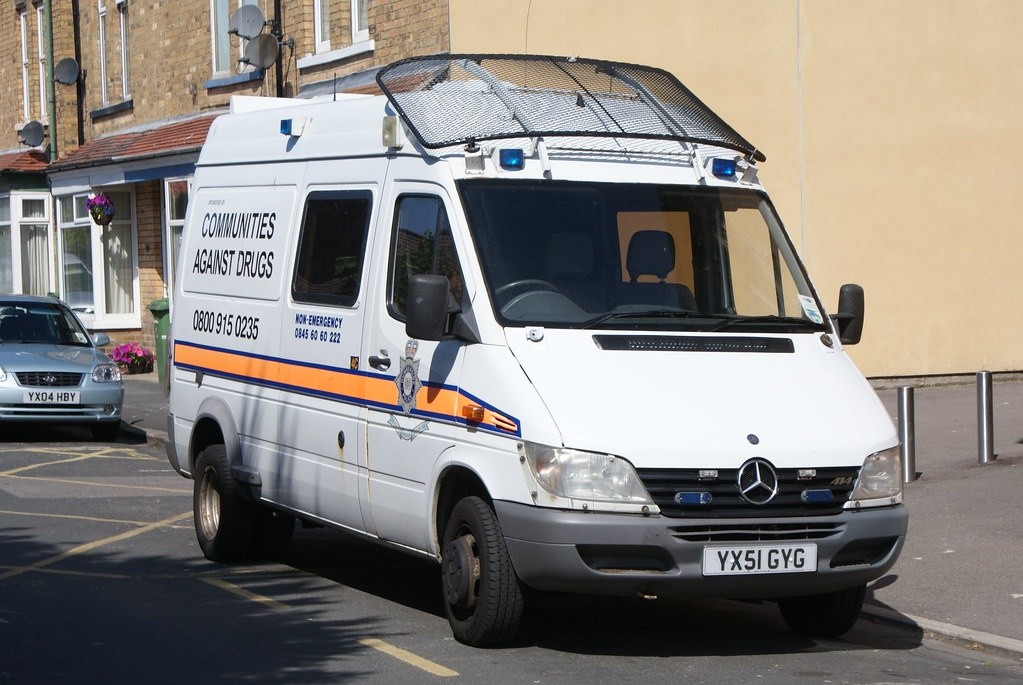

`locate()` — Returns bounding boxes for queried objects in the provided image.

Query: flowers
[112,343,155,365]
[86,192,113,215]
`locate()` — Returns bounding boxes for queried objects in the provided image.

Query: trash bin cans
[144,297,169,386]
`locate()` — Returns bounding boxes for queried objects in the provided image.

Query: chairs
[617,230,699,313]
[534,233,605,314]
[1,316,49,339]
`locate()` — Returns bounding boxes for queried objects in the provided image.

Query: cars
[1,290,123,444]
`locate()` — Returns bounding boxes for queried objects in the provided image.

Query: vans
[173,49,911,651]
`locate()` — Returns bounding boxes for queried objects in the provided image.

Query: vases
[92,214,114,226]
[126,356,148,372]
